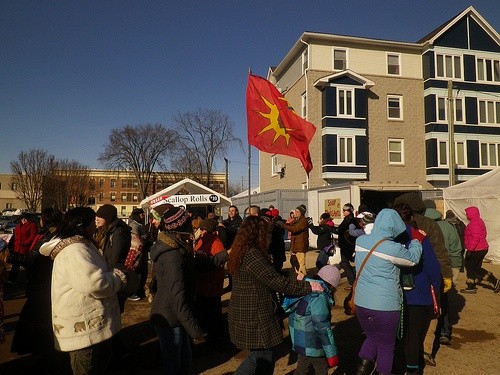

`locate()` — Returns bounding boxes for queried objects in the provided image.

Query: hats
[162,207,192,231]
[200,216,220,232]
[446,210,454,218]
[96,204,118,222]
[260,208,275,218]
[423,199,436,209]
[318,264,340,288]
[357,211,372,218]
[296,204,307,215]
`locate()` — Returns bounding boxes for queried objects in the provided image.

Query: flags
[246,75,317,178]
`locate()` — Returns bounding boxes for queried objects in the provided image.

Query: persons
[149,207,211,375]
[460,206,500,293]
[95,204,132,322]
[127,205,296,300]
[12,208,73,375]
[228,215,324,375]
[392,203,445,375]
[356,204,375,234]
[444,209,467,272]
[3,212,37,300]
[394,192,455,366]
[282,265,340,375]
[421,199,463,344]
[38,205,140,375]
[277,204,309,278]
[307,212,334,270]
[354,208,428,375]
[323,204,362,289]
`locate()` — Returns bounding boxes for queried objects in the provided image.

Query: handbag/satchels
[324,244,335,256]
[343,286,355,315]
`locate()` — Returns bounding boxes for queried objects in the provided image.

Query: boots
[487,273,499,294]
[460,281,477,294]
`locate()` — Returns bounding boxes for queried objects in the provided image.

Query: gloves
[443,276,453,293]
[305,216,313,226]
[276,220,286,227]
[410,227,427,242]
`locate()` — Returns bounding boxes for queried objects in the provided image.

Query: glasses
[343,209,348,212]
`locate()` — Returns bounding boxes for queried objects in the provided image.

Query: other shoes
[421,353,437,366]
[344,284,351,289]
[441,334,453,345]
[125,293,141,301]
